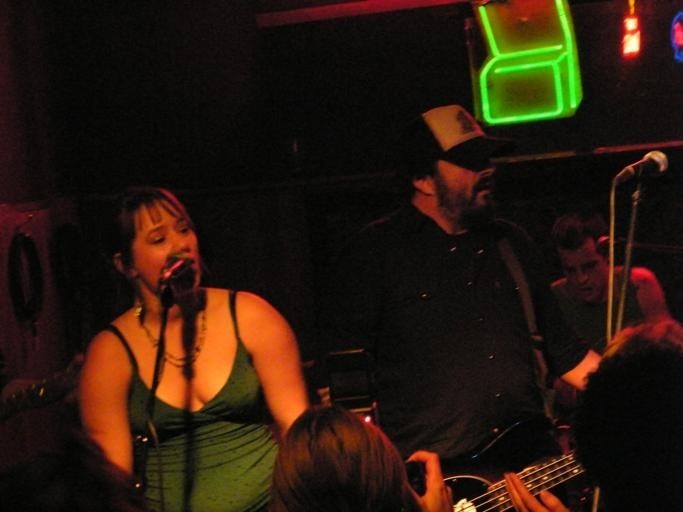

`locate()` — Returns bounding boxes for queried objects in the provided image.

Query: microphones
[616,150,669,184]
[167,255,195,291]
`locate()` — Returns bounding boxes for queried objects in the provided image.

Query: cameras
[325,348,379,427]
[403,458,425,495]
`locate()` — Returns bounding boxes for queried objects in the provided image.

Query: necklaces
[140,306,206,369]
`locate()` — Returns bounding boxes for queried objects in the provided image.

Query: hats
[394,103,514,180]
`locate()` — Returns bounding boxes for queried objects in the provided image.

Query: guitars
[445,449,587,511]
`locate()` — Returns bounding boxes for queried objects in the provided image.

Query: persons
[1,449,144,511]
[271,406,455,511]
[563,335,683,512]
[315,101,552,465]
[77,185,310,511]
[545,211,682,408]
